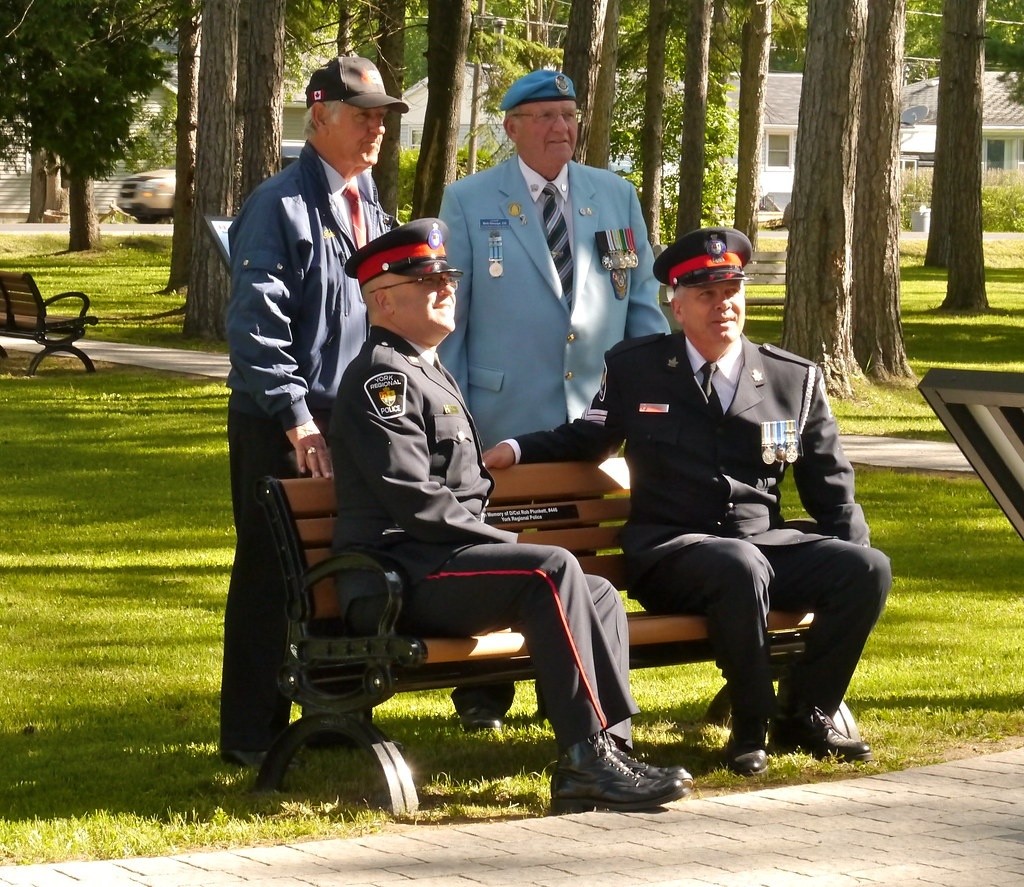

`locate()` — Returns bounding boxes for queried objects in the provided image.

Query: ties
[699,362,722,416]
[541,183,573,310]
[431,351,445,380]
[342,184,363,251]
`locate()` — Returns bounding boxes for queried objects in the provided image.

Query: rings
[307,446,317,454]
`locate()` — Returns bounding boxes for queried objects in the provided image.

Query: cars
[118,139,306,222]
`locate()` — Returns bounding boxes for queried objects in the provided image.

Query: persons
[435,68,674,736]
[328,217,695,814]
[216,55,414,778]
[480,225,893,781]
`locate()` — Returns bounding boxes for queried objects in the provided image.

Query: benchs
[0,270,100,377]
[250,455,819,815]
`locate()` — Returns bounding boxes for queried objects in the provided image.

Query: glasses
[511,110,578,122]
[369,274,459,293]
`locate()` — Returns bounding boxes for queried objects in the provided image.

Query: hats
[306,51,411,114]
[344,217,464,287]
[499,70,576,111]
[651,226,754,289]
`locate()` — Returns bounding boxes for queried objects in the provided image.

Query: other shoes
[462,705,505,733]
[308,729,401,749]
[222,748,296,771]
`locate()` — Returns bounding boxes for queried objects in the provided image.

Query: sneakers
[550,731,693,809]
[773,703,871,761]
[726,718,770,774]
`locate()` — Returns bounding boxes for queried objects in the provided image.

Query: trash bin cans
[910,204,931,232]
